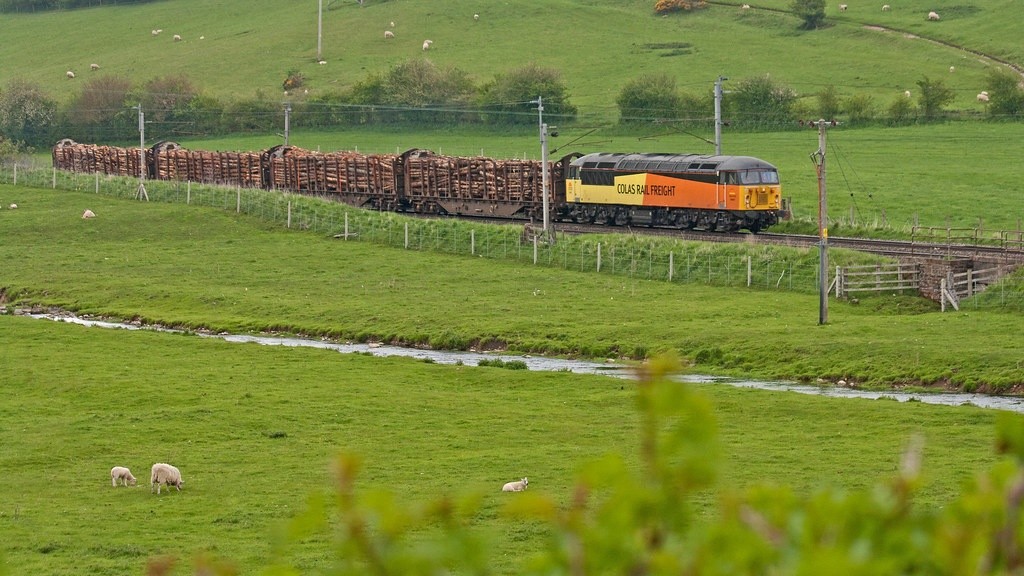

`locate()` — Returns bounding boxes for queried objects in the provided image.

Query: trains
[51,138,786,235]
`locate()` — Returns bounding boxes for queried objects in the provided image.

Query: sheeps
[151,463,185,494]
[111,466,137,487]
[8,5,989,284]
[502,477,528,492]
